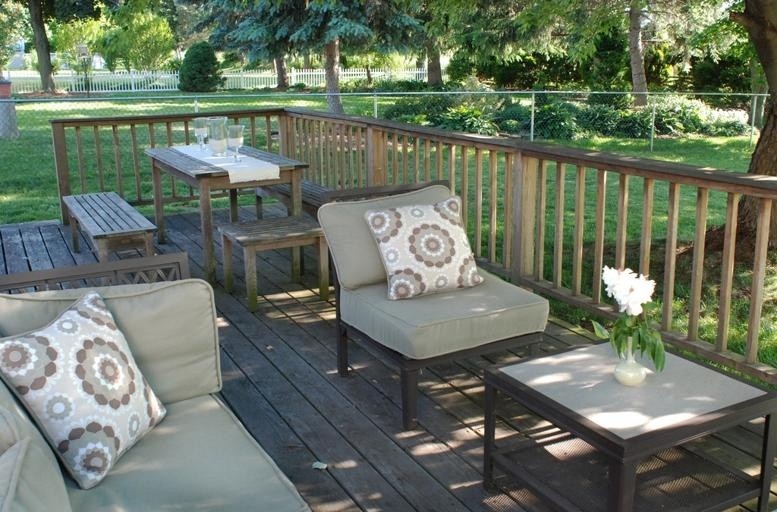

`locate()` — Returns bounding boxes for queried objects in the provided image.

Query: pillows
[1,289,169,491]
[361,190,486,305]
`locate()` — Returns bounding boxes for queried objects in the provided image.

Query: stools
[216,211,331,310]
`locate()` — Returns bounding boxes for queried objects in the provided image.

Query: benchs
[253,178,327,235]
[59,189,169,284]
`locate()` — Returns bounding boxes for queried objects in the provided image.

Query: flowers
[587,263,668,373]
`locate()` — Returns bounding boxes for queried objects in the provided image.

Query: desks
[478,329,777,511]
[142,136,308,284]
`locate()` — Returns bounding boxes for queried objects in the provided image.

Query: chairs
[314,176,553,433]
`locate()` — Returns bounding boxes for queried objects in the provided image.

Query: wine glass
[193,116,210,154]
[227,125,244,164]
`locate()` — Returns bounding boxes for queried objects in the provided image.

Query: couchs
[0,275,312,512]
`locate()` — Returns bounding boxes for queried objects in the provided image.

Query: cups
[206,116,228,154]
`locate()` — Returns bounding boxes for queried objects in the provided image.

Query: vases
[615,334,647,387]
